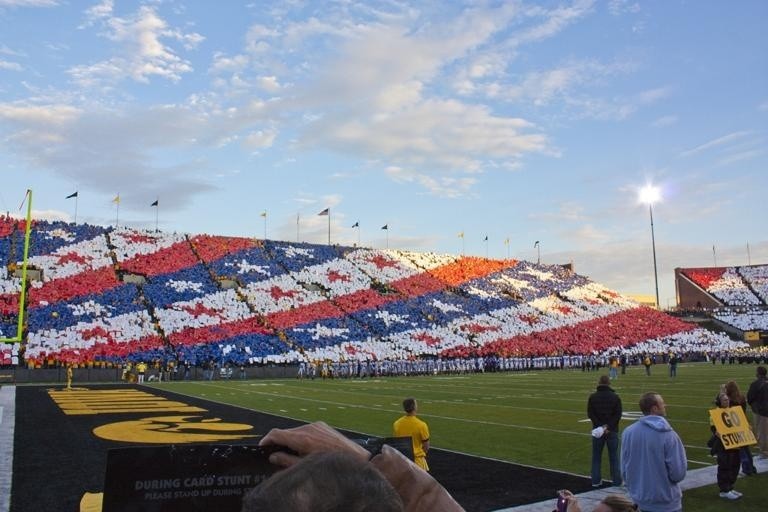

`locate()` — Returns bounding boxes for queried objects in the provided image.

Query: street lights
[636,183,664,308]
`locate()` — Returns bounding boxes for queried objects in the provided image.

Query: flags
[317,208,330,216]
[151,200,158,207]
[113,196,120,203]
[65,191,77,198]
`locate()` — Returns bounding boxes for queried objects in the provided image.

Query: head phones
[716,393,721,406]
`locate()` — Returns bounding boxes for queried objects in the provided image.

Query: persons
[557,489,639,512]
[0,213,678,384]
[720,381,757,475]
[663,266,767,365]
[587,376,622,488]
[241,421,466,512]
[747,366,767,460]
[619,391,688,512]
[707,392,753,501]
[393,399,431,471]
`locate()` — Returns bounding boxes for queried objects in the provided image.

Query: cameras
[557,494,568,512]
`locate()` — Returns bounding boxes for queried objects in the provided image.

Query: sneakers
[719,490,742,499]
[592,480,602,488]
